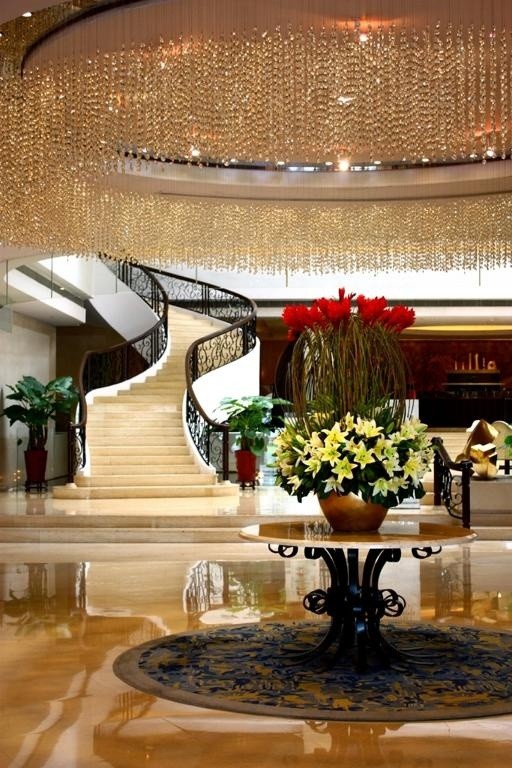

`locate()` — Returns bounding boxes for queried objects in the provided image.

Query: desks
[239,517,479,678]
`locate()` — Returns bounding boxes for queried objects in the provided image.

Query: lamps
[1,0,512,283]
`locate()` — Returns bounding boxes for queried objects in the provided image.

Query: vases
[317,493,390,532]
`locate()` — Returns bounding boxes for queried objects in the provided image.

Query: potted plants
[0,374,79,494]
[213,391,294,490]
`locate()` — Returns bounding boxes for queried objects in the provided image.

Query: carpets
[111,616,511,721]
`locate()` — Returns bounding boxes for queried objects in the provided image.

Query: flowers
[264,286,440,509]
